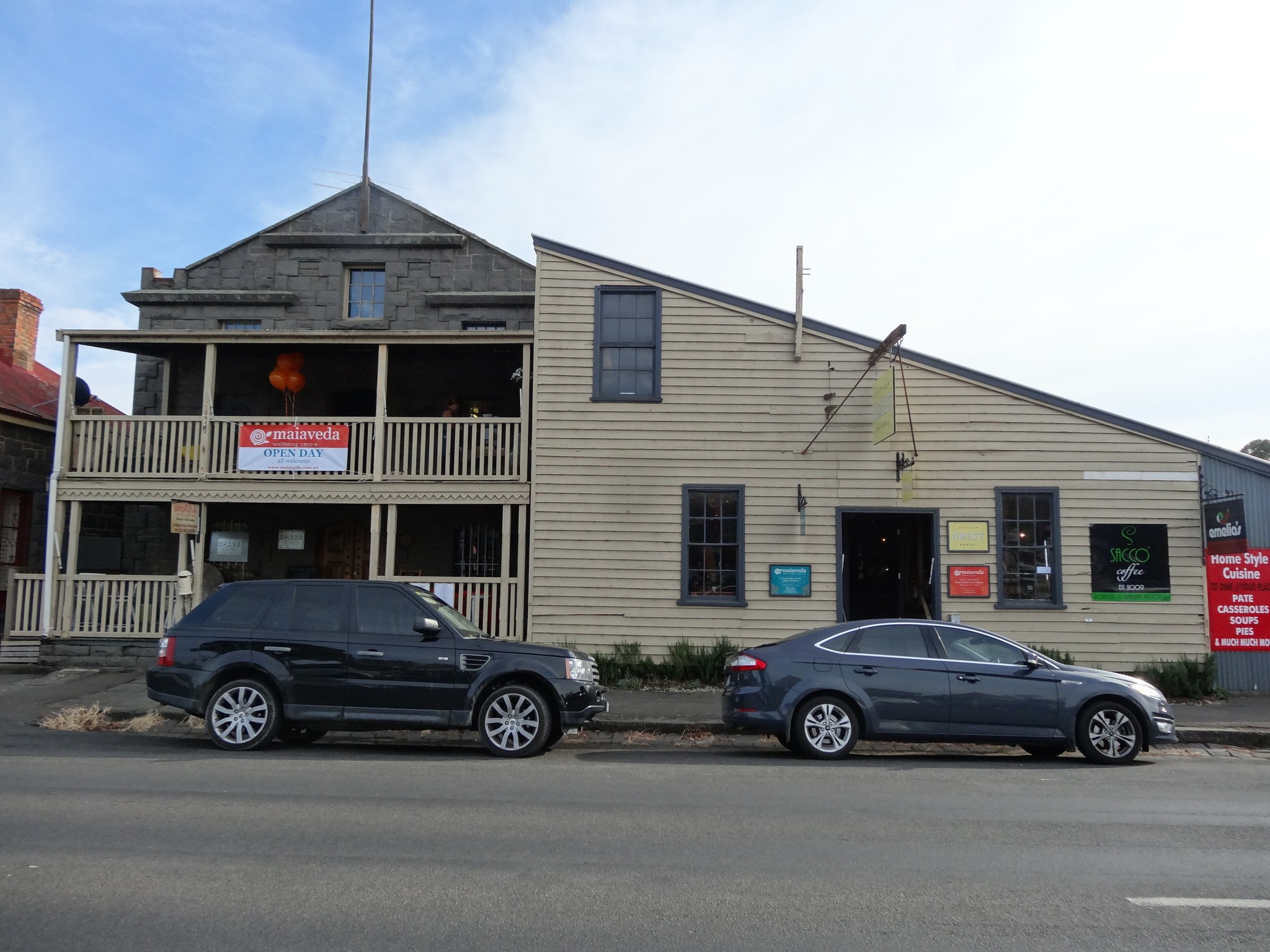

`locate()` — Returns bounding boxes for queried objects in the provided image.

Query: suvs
[146,578,611,759]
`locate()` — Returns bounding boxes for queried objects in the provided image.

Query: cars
[719,618,1179,766]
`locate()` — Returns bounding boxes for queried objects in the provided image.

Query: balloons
[269,352,306,394]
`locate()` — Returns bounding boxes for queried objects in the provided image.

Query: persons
[440,396,464,431]
